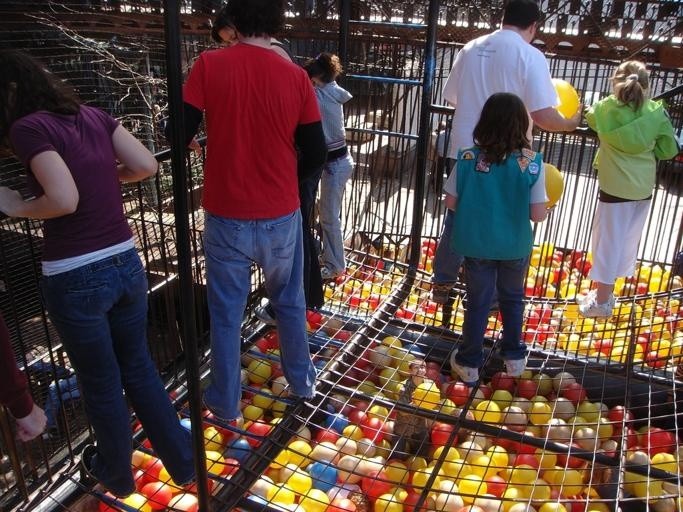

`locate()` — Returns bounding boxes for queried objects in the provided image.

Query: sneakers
[433,288,450,303]
[505,359,525,375]
[81,445,134,498]
[254,305,279,326]
[319,255,343,279]
[450,349,482,383]
[578,289,616,317]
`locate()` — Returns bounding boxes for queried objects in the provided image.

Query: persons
[443,92,551,382]
[573,61,679,318]
[430,1,583,313]
[180,1,357,426]
[1,50,196,500]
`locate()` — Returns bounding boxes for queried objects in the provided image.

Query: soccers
[551,78,580,119]
[541,162,563,206]
